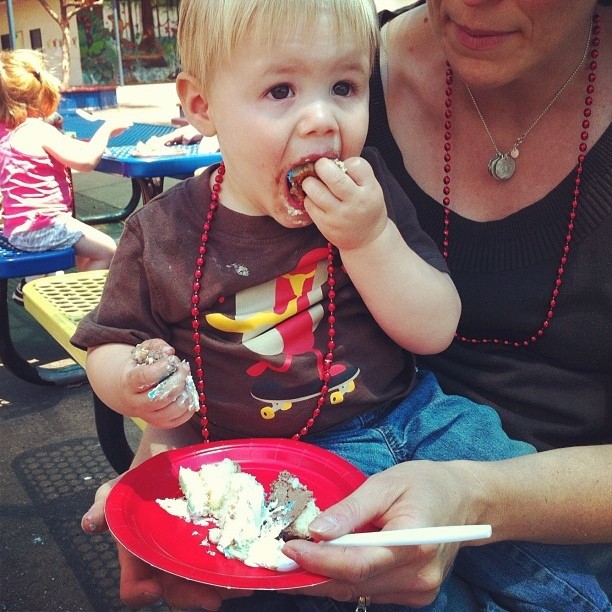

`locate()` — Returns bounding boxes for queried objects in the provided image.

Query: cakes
[155,458,319,572]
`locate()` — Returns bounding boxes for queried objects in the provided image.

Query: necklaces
[192,161,339,447]
[454,17,596,181]
[439,9,603,347]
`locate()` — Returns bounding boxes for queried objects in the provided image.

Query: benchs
[0,244,86,383]
[23,268,147,473]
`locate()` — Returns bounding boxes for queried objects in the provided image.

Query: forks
[255,524,492,572]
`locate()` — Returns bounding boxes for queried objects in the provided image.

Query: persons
[0,47,133,303]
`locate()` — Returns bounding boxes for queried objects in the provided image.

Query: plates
[105,438,369,590]
